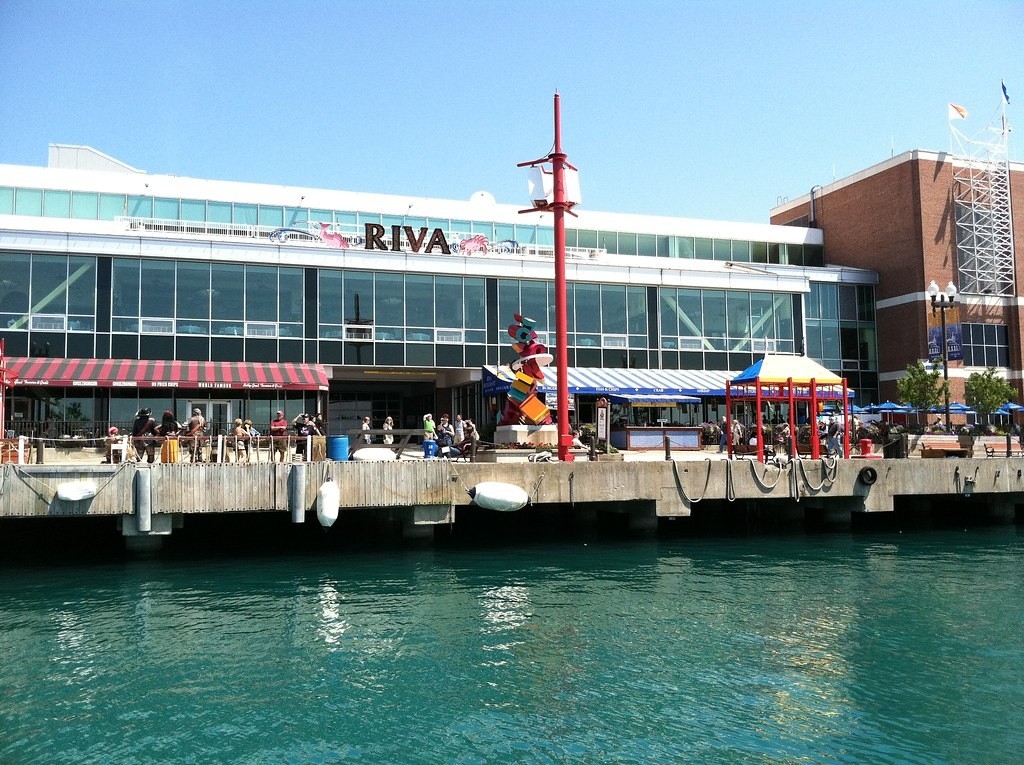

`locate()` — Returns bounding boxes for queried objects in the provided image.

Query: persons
[423,414,437,441]
[818,416,868,458]
[132,408,157,463]
[866,415,1020,435]
[230,419,260,461]
[383,416,394,444]
[182,408,206,463]
[437,413,454,459]
[271,410,288,462]
[362,417,371,444]
[709,413,805,455]
[454,414,480,443]
[154,411,183,463]
[46,417,54,430]
[292,413,327,461]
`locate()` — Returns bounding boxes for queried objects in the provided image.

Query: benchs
[920,441,970,459]
[984,443,1024,457]
[731,445,777,460]
[783,444,829,460]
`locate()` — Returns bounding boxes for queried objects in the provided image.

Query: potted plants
[588,441,624,462]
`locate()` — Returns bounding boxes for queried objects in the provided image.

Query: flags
[949,104,968,120]
[1001,82,1010,104]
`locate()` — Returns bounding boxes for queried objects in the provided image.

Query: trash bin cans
[328,436,349,460]
[883,433,908,459]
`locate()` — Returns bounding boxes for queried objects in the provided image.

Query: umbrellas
[817,401,1024,426]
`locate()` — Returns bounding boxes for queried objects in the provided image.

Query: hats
[276,411,284,416]
[134,407,152,417]
[302,413,309,418]
[193,408,202,414]
[109,426,118,435]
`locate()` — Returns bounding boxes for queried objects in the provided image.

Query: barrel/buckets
[326,435,350,461]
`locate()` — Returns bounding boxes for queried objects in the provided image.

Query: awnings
[0,356,329,391]
[482,366,855,398]
